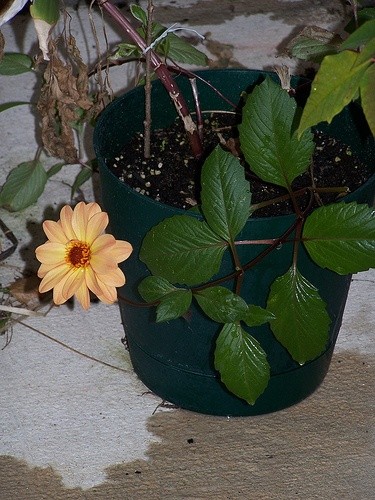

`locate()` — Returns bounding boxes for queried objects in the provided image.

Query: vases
[94,69,374,416]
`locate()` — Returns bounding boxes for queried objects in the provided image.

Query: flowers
[1,2,374,406]
